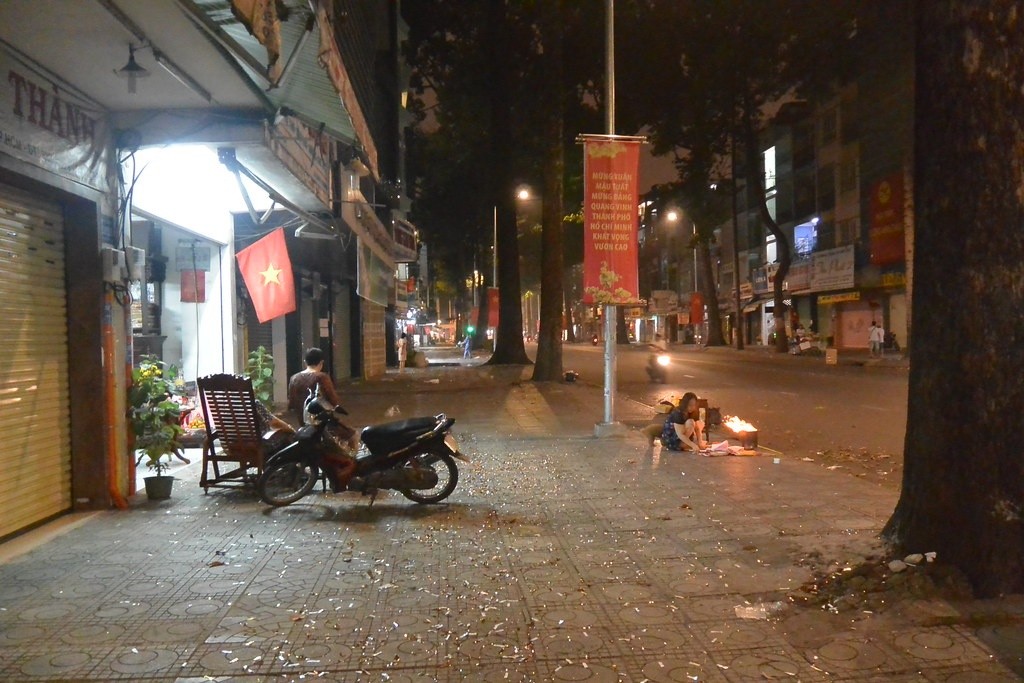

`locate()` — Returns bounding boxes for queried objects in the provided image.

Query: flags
[238,227,296,324]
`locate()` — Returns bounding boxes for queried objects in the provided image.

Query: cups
[773,458,781,464]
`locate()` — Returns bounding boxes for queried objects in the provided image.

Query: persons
[877,324,884,358]
[462,333,471,359]
[869,322,880,359]
[661,392,708,454]
[797,325,805,333]
[649,333,666,353]
[397,333,407,373]
[228,388,296,452]
[289,347,359,451]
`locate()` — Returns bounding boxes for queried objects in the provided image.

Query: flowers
[133,356,190,475]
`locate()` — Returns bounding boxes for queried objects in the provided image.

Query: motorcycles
[873,330,900,352]
[645,343,672,384]
[256,383,471,506]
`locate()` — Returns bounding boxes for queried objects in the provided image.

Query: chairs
[196,374,285,496]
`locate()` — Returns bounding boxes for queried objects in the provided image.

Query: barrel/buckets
[708,407,721,425]
[826,348,837,364]
[741,430,758,451]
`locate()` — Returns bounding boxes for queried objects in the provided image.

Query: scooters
[592,337,598,346]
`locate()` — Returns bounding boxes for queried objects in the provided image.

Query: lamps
[342,149,371,176]
[117,50,149,97]
[154,52,213,103]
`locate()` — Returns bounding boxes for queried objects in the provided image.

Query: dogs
[641,424,694,447]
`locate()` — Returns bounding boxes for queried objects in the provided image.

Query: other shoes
[675,445,691,452]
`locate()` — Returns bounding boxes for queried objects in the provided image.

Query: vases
[144,476,173,499]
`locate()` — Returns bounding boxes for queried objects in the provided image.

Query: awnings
[185,1,379,183]
[0,0,275,119]
